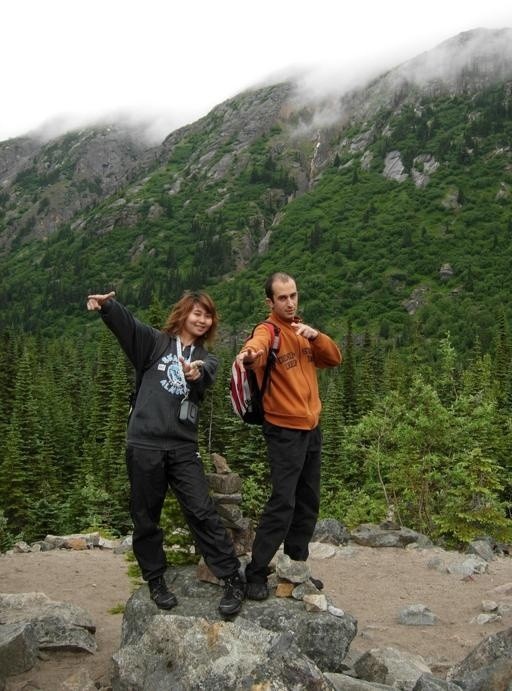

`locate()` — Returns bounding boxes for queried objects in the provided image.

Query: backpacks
[127,333,208,426]
[230,321,282,424]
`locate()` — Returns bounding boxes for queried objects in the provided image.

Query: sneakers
[219,570,268,615]
[309,576,323,589]
[147,575,178,610]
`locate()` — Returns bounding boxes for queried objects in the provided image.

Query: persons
[235,271,343,601]
[86,291,247,616]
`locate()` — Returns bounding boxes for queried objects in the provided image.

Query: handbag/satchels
[179,401,198,425]
